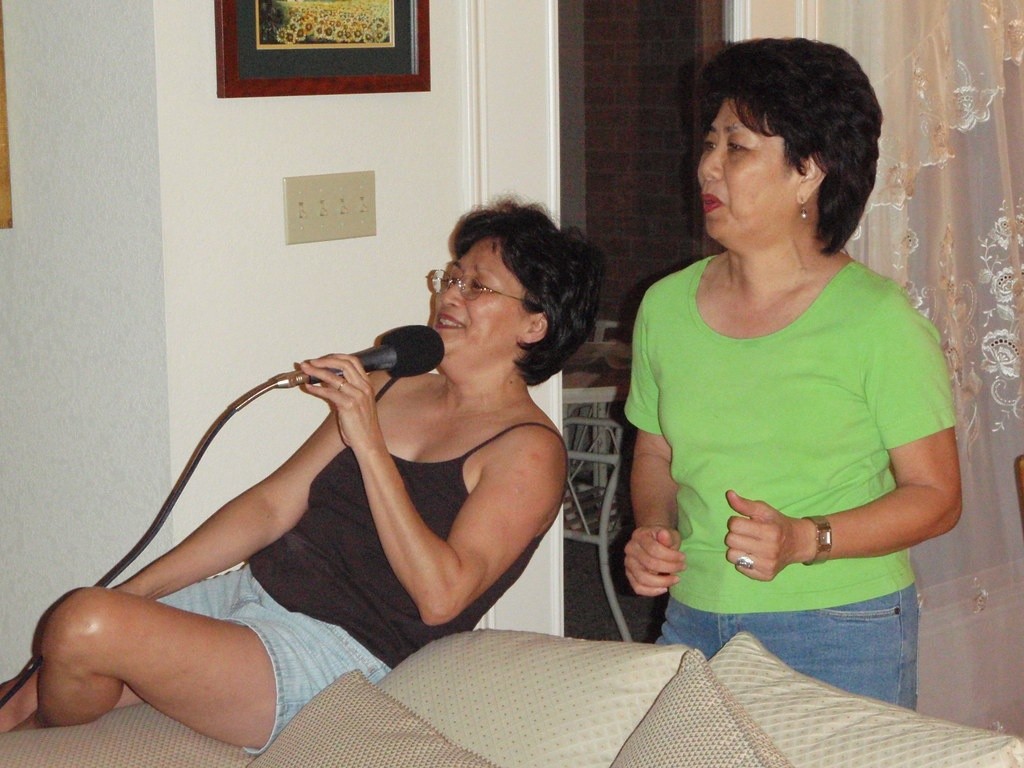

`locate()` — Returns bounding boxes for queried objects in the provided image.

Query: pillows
[247,624,1024,767]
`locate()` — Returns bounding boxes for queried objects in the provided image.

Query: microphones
[275,324,445,389]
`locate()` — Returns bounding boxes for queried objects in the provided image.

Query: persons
[624,37,962,712]
[1,198,605,755]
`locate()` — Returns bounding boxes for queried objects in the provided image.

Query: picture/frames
[214,0,431,98]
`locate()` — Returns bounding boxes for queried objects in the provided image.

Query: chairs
[563,416,635,642]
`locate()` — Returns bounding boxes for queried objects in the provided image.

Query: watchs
[802,516,832,565]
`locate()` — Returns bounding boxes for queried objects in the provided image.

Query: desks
[563,339,631,529]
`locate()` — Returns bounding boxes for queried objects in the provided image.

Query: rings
[737,553,754,569]
[337,380,347,393]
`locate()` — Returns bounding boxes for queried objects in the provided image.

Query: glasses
[427,269,543,310]
